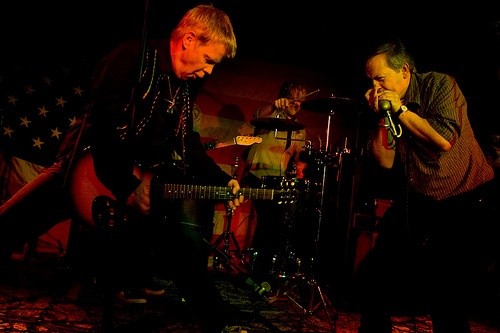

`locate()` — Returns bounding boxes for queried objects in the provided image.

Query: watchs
[394,105,408,118]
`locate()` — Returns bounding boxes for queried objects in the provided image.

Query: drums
[208,148,329,270]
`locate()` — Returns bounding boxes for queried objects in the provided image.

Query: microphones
[380,100,398,138]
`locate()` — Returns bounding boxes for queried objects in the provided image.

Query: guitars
[73,153,300,225]
[202,136,263,150]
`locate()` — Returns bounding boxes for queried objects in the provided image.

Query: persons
[240,82,306,275]
[1,4,243,332]
[358,43,500,333]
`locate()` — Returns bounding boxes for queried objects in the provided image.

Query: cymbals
[251,116,304,133]
[301,98,359,114]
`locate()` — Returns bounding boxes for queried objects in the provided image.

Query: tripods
[213,110,339,331]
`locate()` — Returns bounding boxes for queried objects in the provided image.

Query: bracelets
[379,123,390,127]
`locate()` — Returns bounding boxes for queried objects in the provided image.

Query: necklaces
[167,76,180,110]
